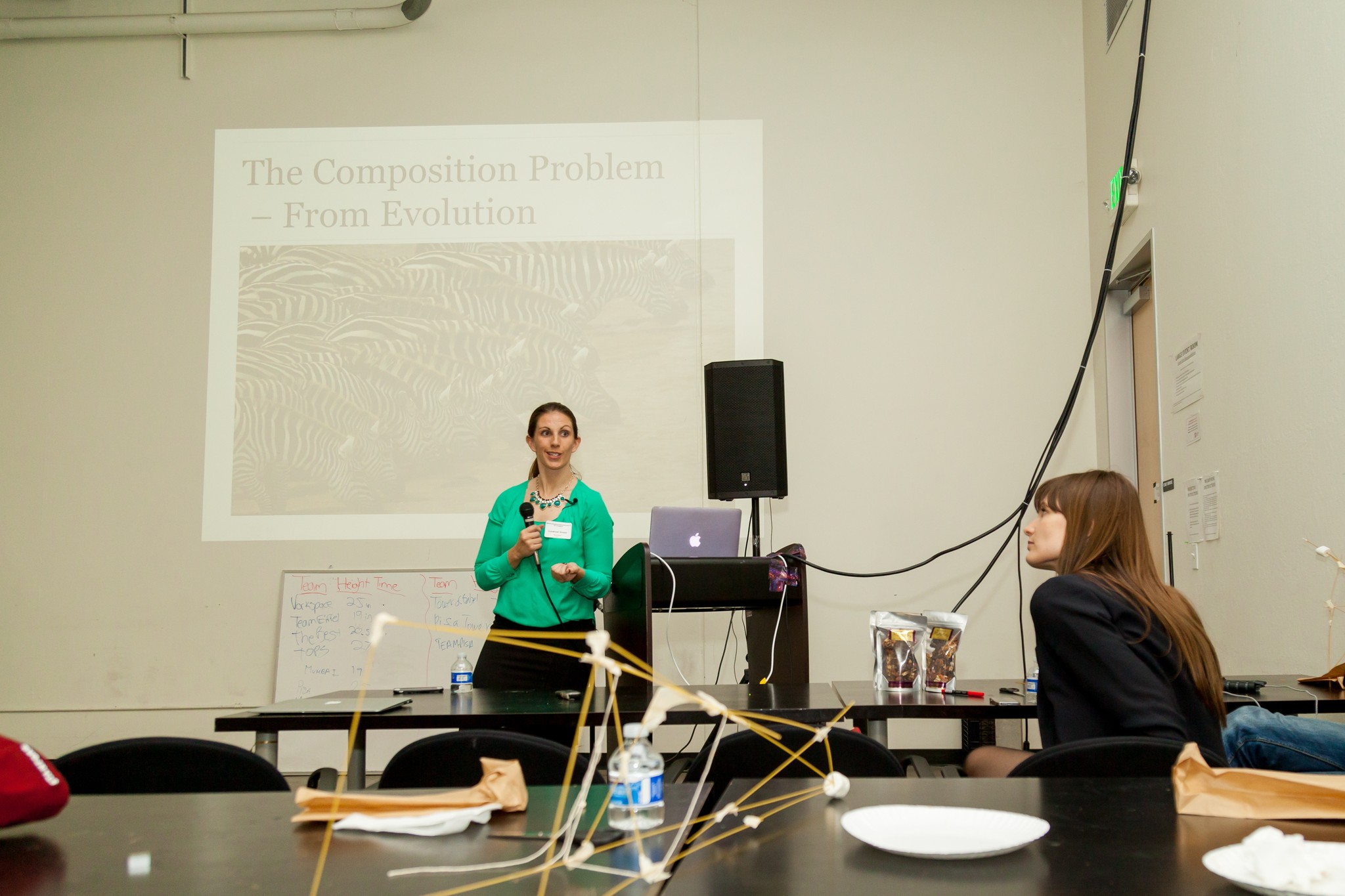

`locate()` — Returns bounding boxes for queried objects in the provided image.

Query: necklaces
[529,474,574,510]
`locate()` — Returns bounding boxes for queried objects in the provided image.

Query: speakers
[704,359,788,500]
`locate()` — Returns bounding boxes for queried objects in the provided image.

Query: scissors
[487,830,625,846]
[999,688,1025,696]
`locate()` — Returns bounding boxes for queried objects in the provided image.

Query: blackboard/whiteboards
[271,569,500,775]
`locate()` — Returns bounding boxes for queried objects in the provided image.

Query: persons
[965,469,1228,778]
[1220,705,1345,773]
[474,402,614,748]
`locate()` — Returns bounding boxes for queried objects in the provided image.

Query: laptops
[244,697,413,714]
[648,506,742,558]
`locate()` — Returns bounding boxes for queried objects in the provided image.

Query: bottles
[450,654,473,694]
[606,723,665,832]
[1026,671,1038,702]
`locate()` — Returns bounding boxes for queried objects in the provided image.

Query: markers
[942,690,984,697]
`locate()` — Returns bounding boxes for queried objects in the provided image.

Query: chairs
[52,737,291,794]
[683,724,902,783]
[379,729,605,788]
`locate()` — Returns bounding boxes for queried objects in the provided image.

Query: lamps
[1103,158,1141,226]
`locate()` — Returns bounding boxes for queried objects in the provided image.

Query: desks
[0,676,1345,896]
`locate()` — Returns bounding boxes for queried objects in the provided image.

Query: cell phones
[393,686,443,695]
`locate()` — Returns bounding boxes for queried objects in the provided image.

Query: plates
[1201,841,1345,896]
[840,804,1050,859]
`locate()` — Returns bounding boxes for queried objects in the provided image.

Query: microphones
[519,502,541,571]
[565,498,578,505]
